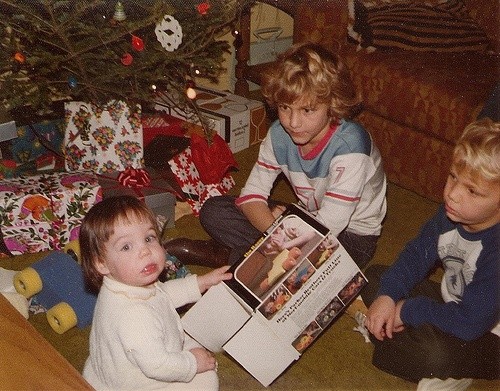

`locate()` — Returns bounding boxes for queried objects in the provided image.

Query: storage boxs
[155,80,232,124]
[102,167,182,238]
[0,170,104,257]
[192,93,270,156]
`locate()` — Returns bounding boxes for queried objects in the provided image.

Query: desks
[0,293,96,391]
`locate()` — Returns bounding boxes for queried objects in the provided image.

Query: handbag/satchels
[64,97,144,175]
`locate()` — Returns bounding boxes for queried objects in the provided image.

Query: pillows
[359,4,496,60]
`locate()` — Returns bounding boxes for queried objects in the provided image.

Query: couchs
[292,0,500,222]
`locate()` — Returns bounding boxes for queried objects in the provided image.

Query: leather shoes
[163,238,231,268]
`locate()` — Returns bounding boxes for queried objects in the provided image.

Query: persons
[256,217,315,255]
[77,194,233,391]
[344,118,500,391]
[163,42,387,271]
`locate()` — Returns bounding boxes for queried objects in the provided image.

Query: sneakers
[344,295,371,343]
[417,374,473,391]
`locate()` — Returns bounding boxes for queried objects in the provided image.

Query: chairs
[235,0,305,117]
